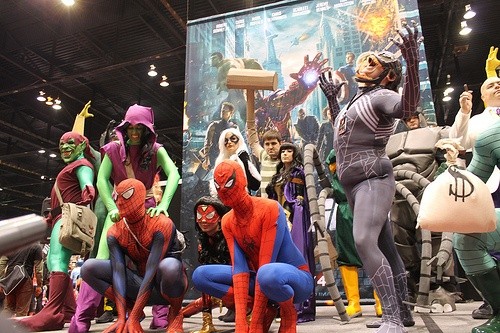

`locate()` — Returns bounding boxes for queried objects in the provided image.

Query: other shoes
[218,308,252,322]
[472,302,492,318]
[96,311,114,323]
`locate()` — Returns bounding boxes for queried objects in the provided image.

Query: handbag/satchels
[59,203,97,258]
[0,265,29,296]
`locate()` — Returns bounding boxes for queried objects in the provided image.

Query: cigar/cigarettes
[464,84,468,92]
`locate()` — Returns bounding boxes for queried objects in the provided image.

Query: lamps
[463,4,477,19]
[459,20,473,35]
[441,74,454,101]
[37,88,62,109]
[159,74,169,87]
[147,64,158,77]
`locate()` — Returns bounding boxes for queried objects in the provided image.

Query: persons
[265,142,316,323]
[20,132,96,332]
[316,105,334,161]
[295,109,319,144]
[71,261,83,292]
[80,178,188,333]
[435,123,500,333]
[243,51,330,143]
[215,128,261,196]
[448,76,500,208]
[181,196,232,333]
[385,106,441,306]
[318,23,424,333]
[211,52,264,123]
[69,105,180,333]
[191,160,313,333]
[326,148,382,318]
[72,100,121,258]
[245,88,282,197]
[195,102,238,179]
[0,240,50,317]
[338,50,358,98]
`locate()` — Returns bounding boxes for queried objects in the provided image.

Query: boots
[373,289,383,317]
[338,266,362,319]
[466,266,500,333]
